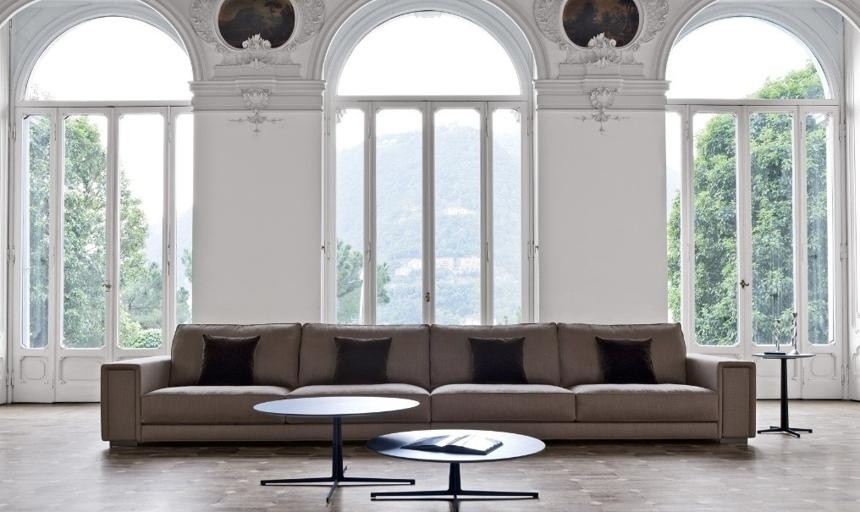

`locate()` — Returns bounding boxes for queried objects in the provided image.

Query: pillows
[333,336,392,385]
[194,334,261,386]
[467,337,529,384]
[595,336,659,384]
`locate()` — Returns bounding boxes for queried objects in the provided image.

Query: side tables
[753,353,815,438]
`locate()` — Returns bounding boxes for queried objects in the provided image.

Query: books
[400,435,503,455]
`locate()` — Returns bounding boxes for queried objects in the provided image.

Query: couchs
[100,322,757,448]
